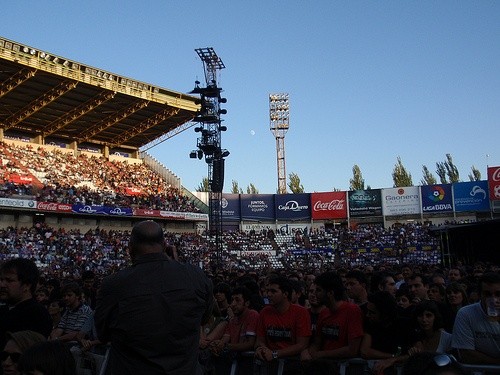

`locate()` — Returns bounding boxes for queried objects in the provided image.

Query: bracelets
[391,353,395,358]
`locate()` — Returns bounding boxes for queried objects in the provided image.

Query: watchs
[272,350,277,359]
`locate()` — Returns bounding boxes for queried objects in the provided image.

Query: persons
[404,349,460,375]
[3,331,46,373]
[95,219,215,375]
[255,277,310,361]
[216,286,259,353]
[0,219,500,322]
[18,341,75,374]
[453,272,500,365]
[197,310,227,349]
[300,269,362,363]
[1,259,53,339]
[1,137,199,212]
[401,300,452,354]
[360,289,414,371]
[70,307,97,374]
[48,282,94,342]
